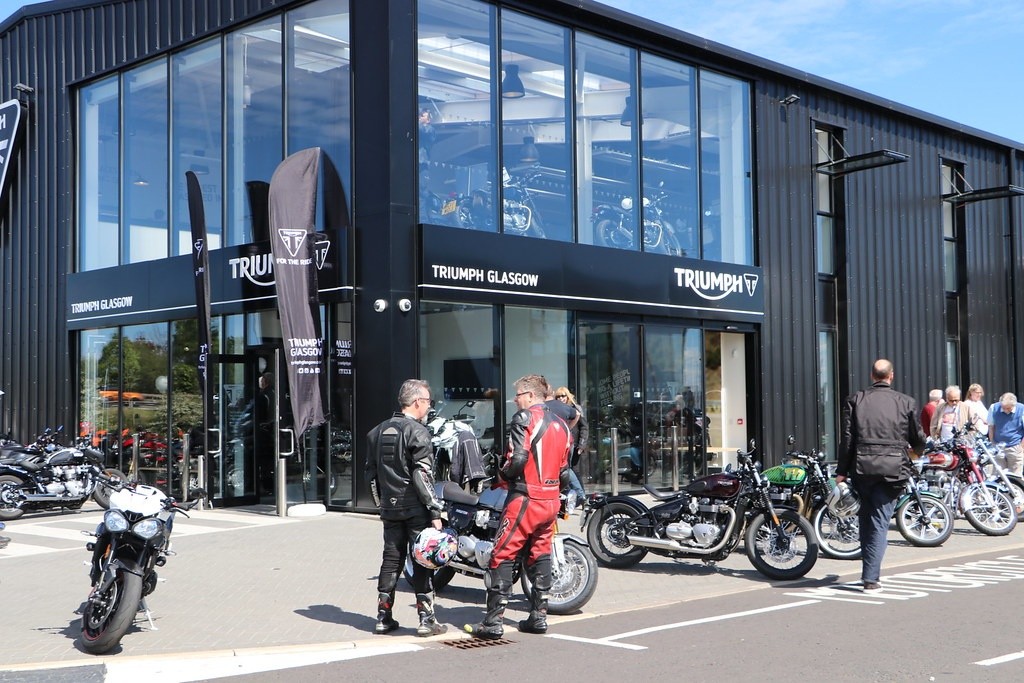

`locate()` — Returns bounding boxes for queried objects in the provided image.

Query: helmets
[411,526,459,570]
[826,481,861,517]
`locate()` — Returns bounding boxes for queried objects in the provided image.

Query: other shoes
[861,577,883,593]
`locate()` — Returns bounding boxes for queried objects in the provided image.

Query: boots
[416,590,448,637]
[375,589,399,633]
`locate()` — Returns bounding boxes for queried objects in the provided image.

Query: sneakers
[519,610,548,633]
[464,618,504,640]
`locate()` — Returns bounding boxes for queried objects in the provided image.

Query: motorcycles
[725,435,864,562]
[401,466,603,614]
[298,423,351,498]
[592,403,706,486]
[580,436,819,581]
[0,424,125,521]
[80,420,207,498]
[829,421,1024,548]
[424,400,515,488]
[79,447,207,655]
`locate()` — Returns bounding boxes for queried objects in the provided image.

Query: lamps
[780,94,800,105]
[621,97,644,127]
[243,38,251,106]
[133,175,148,186]
[501,65,525,99]
[112,122,136,136]
[518,135,540,163]
[188,149,209,175]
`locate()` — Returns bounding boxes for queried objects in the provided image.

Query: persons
[364,378,447,637]
[542,384,594,513]
[418,109,437,224]
[464,375,574,640]
[919,383,1024,478]
[234,367,289,498]
[834,358,927,595]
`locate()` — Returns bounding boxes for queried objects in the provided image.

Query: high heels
[576,495,586,510]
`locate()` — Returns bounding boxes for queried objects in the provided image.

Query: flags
[267,147,336,439]
[184,171,213,429]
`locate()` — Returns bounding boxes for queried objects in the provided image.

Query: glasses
[948,397,958,402]
[556,395,567,398]
